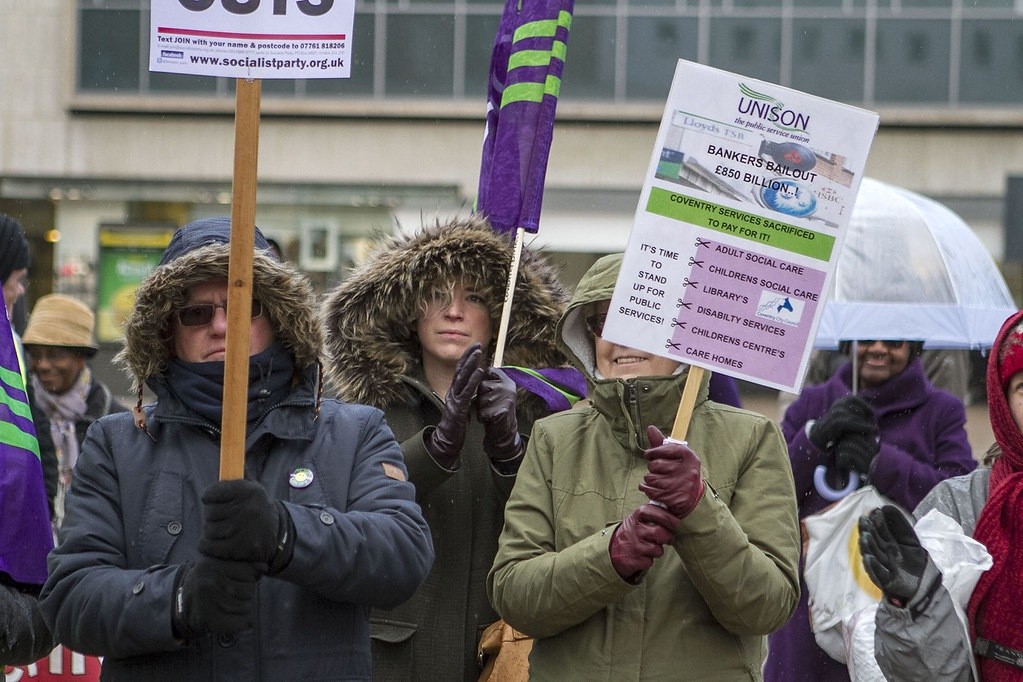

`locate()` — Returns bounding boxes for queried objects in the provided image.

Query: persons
[0,217,1023,682]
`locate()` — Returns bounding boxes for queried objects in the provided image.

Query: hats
[21,291,100,360]
[996,319,1023,388]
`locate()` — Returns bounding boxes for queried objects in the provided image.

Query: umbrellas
[808,183,1016,350]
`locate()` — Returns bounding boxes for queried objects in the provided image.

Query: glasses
[174,296,263,329]
[584,313,607,340]
[857,337,905,349]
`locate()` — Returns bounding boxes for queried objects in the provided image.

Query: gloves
[427,340,486,471]
[172,557,253,638]
[808,393,879,452]
[609,505,681,586]
[636,424,704,520]
[474,368,523,465]
[837,433,878,488]
[197,470,294,580]
[859,503,927,606]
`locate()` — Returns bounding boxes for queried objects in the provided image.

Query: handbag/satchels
[800,490,914,662]
[917,508,994,682]
